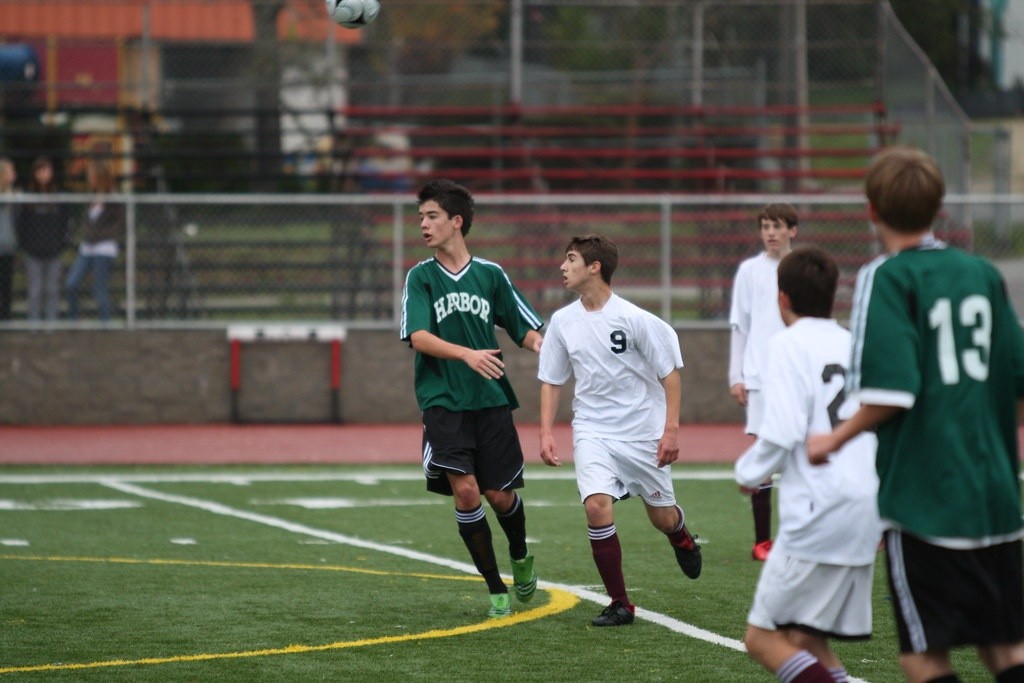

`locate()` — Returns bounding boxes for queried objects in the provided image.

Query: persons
[726,203,798,561]
[807,149,1024,683]
[400,178,544,618]
[732,248,884,683]
[538,233,701,626]
[0,154,121,319]
[728,202,798,561]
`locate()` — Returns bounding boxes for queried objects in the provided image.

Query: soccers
[326,0,383,31]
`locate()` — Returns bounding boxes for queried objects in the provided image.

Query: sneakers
[753,541,772,561]
[487,594,510,619]
[667,525,702,580]
[509,542,536,603]
[592,598,635,627]
